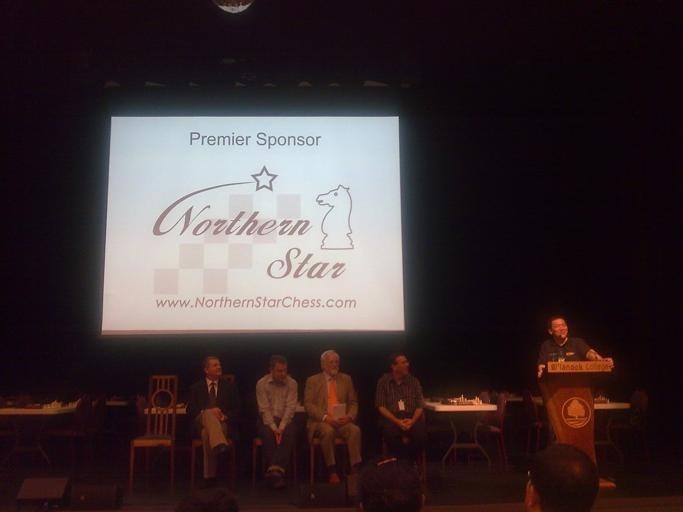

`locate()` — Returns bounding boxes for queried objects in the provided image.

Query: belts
[347,475,360,498]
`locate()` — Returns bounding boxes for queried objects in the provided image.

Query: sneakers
[264,466,286,487]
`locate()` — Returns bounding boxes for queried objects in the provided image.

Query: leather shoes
[330,473,339,486]
[214,445,229,479]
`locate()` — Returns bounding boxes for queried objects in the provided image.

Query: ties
[210,383,215,399]
[329,379,335,416]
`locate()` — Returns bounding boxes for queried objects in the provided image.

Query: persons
[524,443,599,512]
[303,350,362,486]
[255,354,298,488]
[175,479,239,512]
[375,352,426,456]
[537,315,615,378]
[360,456,425,512]
[188,354,237,489]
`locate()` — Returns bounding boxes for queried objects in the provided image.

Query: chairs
[608,387,651,464]
[472,389,546,465]
[127,375,239,495]
[44,394,107,466]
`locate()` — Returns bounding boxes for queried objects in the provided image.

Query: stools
[251,437,298,485]
[309,437,363,497]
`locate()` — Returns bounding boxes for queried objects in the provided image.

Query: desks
[424,396,633,470]
[0,398,129,471]
